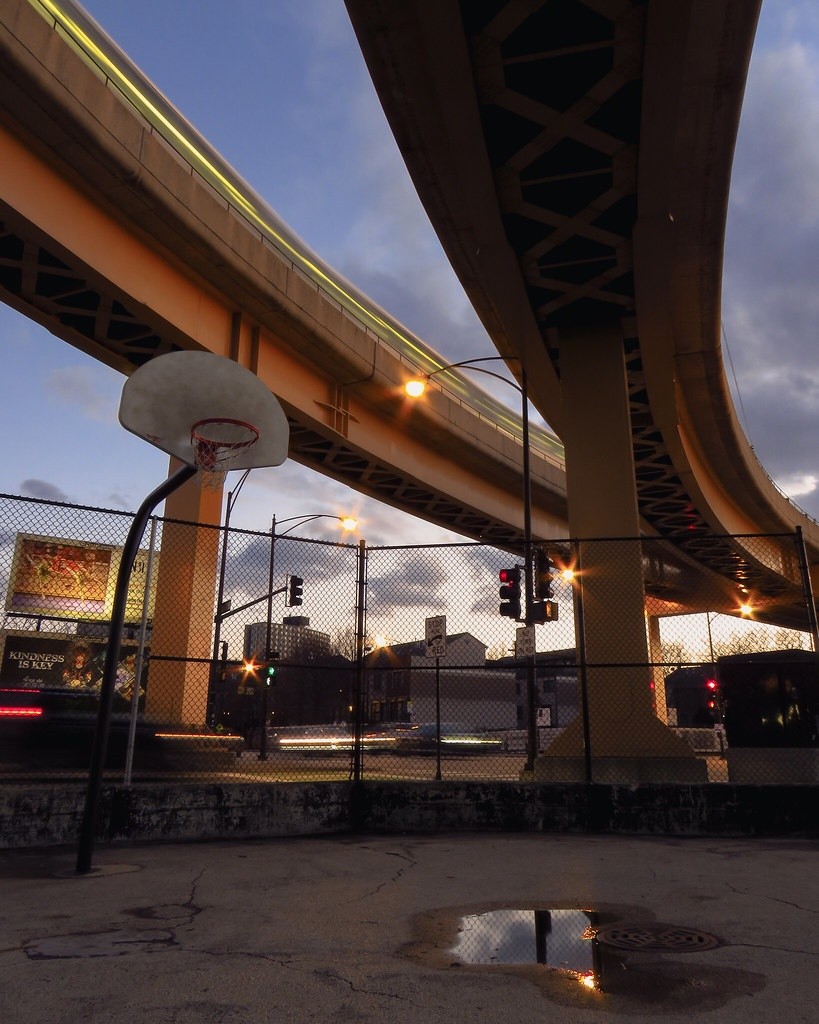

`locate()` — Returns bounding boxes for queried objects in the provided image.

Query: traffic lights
[707,700,717,711]
[291,574,303,606]
[708,681,716,688]
[267,666,276,677]
[533,549,556,599]
[498,566,520,621]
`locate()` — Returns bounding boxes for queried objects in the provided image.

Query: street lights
[405,355,540,773]
[564,567,592,784]
[256,514,360,759]
[706,606,757,724]
